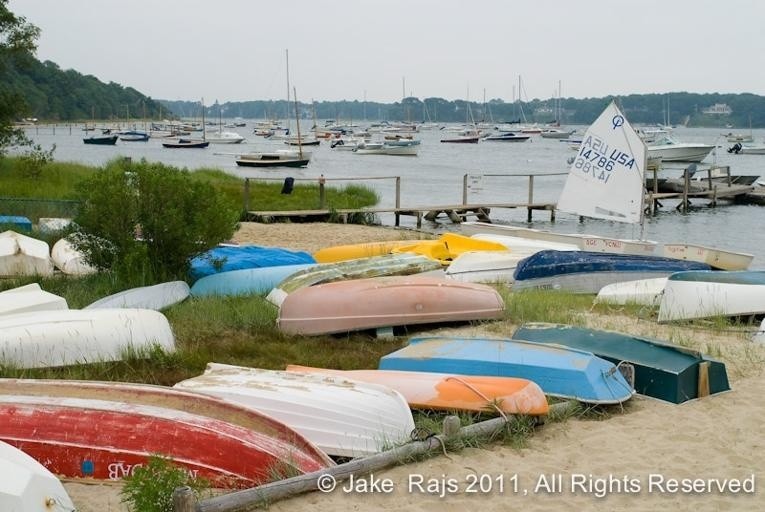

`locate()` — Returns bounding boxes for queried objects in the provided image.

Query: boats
[632,125,716,162]
[721,131,765,155]
[645,168,765,210]
